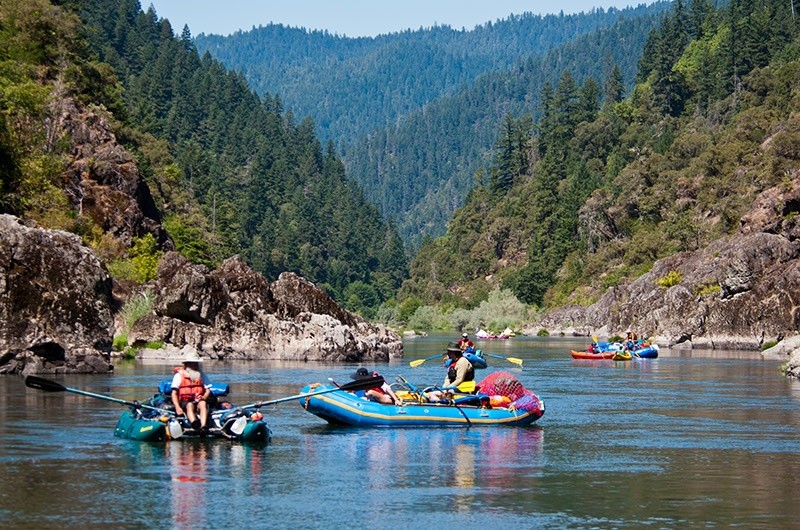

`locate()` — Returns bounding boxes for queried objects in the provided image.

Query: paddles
[208,375,385,415]
[24,374,177,416]
[431,380,475,392]
[483,353,523,365]
[592,335,606,360]
[410,353,448,367]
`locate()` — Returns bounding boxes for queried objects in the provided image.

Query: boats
[114,392,270,450]
[444,353,488,369]
[570,346,658,361]
[479,333,515,339]
[298,381,547,426]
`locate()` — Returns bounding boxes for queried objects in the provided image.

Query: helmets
[591,342,596,345]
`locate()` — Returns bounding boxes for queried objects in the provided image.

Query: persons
[587,332,651,356]
[440,344,476,394]
[487,331,494,336]
[171,353,210,438]
[349,368,402,407]
[456,333,474,353]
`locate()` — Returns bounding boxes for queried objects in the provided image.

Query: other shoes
[191,419,203,435]
[199,426,208,442]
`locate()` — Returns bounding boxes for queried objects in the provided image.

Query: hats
[180,347,203,362]
[350,368,372,380]
[442,342,462,351]
[462,332,468,337]
